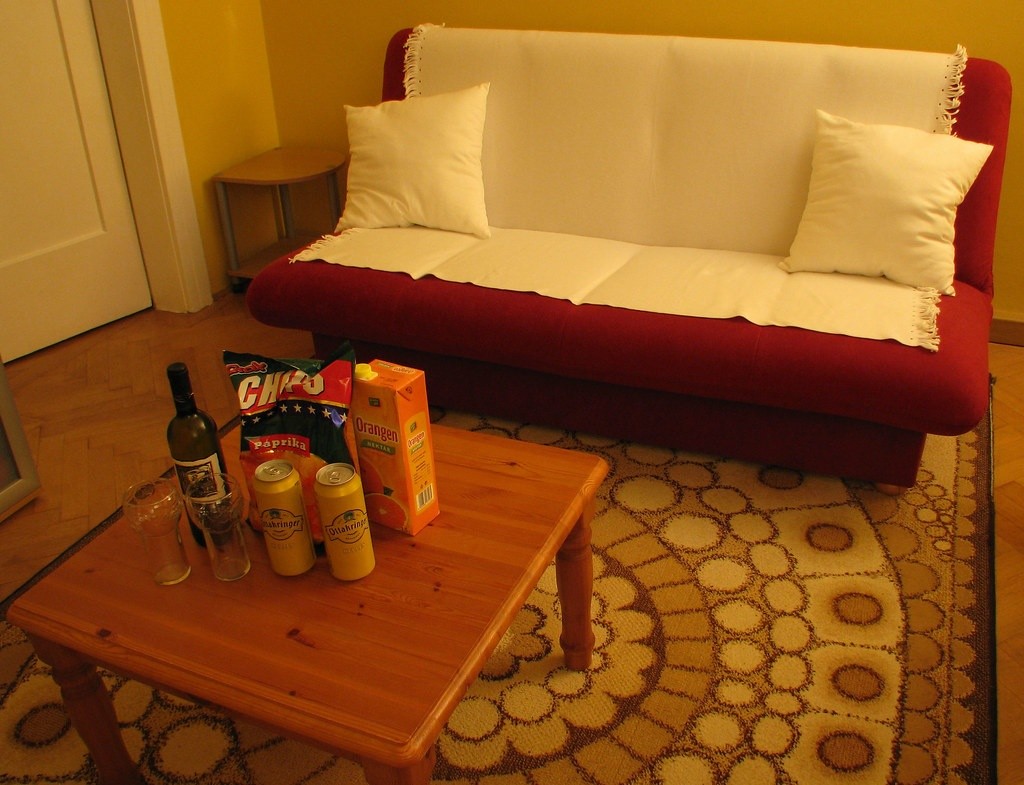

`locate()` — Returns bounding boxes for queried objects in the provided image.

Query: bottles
[166,363,232,547]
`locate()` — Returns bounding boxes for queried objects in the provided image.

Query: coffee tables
[5,420,609,785]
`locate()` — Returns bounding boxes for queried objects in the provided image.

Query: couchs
[244,21,1013,494]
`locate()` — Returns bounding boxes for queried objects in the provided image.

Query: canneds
[252,459,316,576]
[314,463,375,581]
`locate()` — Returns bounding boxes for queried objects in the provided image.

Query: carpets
[0,352,998,785]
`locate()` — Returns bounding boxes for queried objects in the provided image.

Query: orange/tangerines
[358,457,407,531]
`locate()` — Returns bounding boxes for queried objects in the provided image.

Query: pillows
[335,82,489,239]
[778,107,993,297]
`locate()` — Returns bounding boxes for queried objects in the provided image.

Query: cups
[121,477,189,586]
[187,473,249,582]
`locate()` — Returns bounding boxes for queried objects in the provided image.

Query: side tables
[215,146,346,293]
